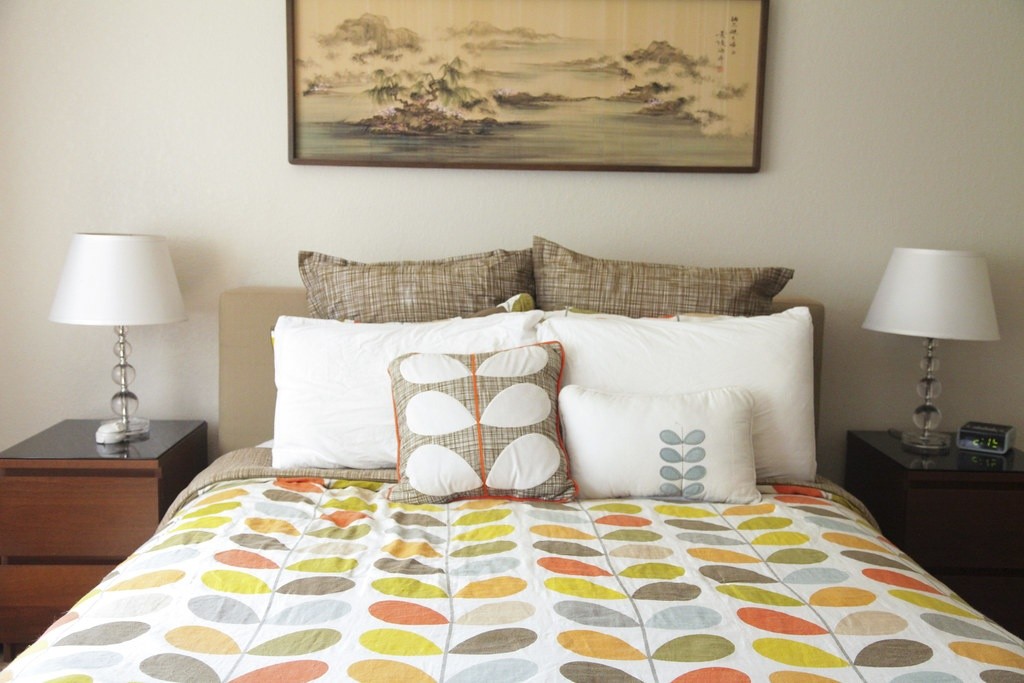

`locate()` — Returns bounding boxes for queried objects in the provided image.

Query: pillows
[270,293,535,350]
[297,247,534,323]
[538,306,818,484]
[532,235,796,318]
[272,310,546,469]
[558,385,762,507]
[387,340,580,505]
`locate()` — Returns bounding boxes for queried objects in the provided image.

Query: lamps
[861,246,1002,455]
[49,233,190,444]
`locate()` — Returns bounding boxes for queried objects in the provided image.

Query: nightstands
[842,428,1024,641]
[0,419,208,663]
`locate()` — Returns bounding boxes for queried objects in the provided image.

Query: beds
[0,286,1024,683]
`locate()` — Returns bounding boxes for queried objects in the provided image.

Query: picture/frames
[285,0,770,175]
